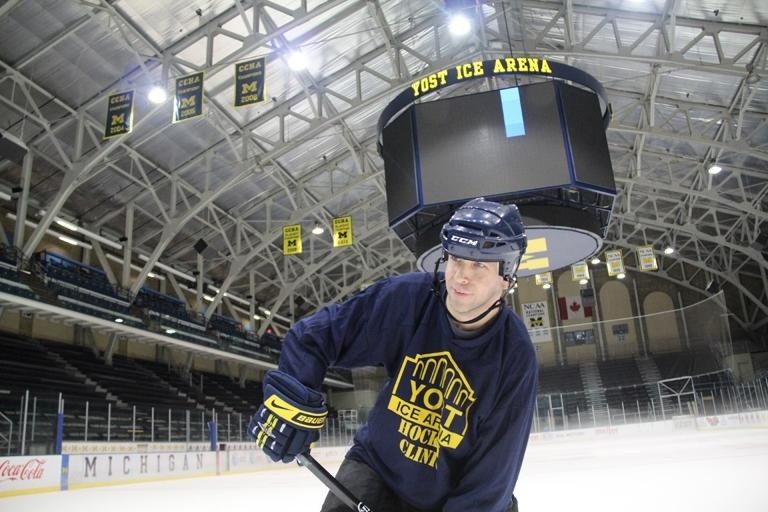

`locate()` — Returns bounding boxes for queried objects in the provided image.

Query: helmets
[441,197,526,282]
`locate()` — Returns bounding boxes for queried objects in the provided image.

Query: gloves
[247,370,328,464]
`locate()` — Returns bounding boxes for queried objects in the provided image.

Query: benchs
[0,330,264,454]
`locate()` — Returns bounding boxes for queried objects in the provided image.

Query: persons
[246,198,542,512]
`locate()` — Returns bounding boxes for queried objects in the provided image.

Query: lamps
[312,221,324,234]
[665,243,673,254]
[709,158,721,174]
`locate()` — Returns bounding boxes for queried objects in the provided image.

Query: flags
[101,88,135,138]
[231,57,270,108]
[535,270,554,286]
[173,69,207,123]
[280,224,304,256]
[603,249,627,276]
[331,214,353,247]
[634,243,660,273]
[570,262,590,281]
